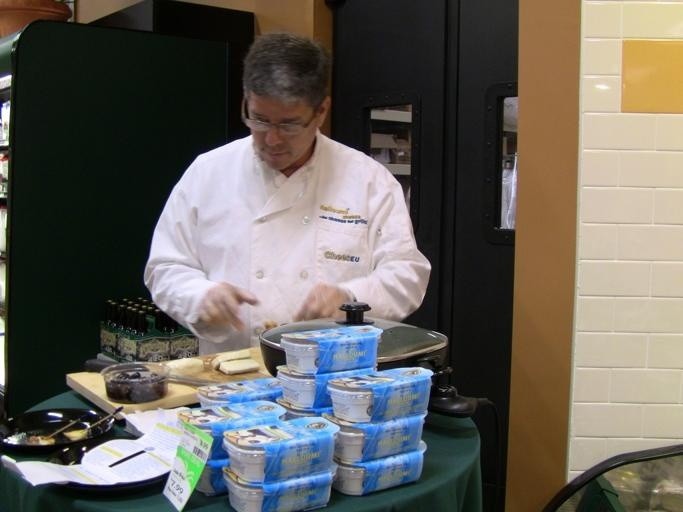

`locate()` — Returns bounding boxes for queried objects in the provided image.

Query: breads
[167,350,262,376]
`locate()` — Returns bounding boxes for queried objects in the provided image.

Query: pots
[258,302,450,381]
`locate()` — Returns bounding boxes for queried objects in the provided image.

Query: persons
[142,32,433,355]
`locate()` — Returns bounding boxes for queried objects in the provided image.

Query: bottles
[99,296,181,361]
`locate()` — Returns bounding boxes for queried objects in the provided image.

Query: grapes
[106,369,165,403]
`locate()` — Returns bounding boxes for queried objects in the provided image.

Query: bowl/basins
[0,406,111,457]
[103,361,169,403]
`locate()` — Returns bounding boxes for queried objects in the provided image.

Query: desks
[1,391,484,512]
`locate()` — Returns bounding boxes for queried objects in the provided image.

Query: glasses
[242,98,320,135]
